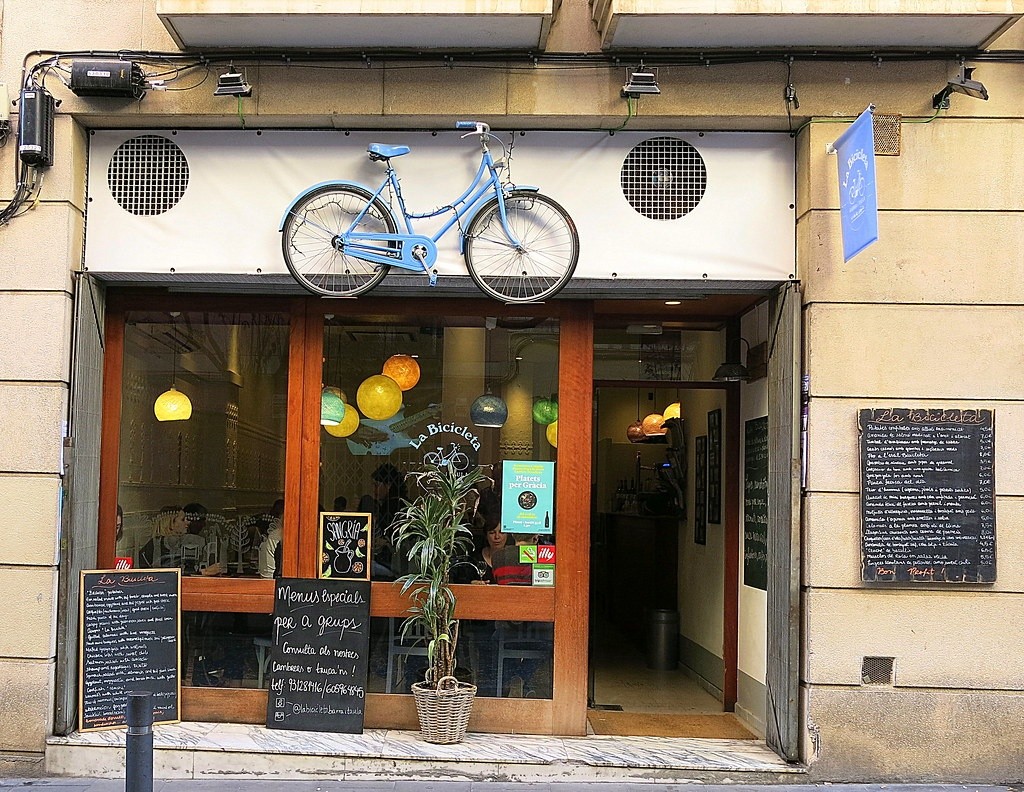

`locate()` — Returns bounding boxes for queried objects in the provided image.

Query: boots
[192,656,219,686]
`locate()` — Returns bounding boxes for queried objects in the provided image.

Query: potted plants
[384,444,495,744]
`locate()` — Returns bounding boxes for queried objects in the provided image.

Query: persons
[317,463,556,699]
[116,503,123,542]
[138,500,284,687]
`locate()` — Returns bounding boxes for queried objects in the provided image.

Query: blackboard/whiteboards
[858,408,998,583]
[743,413,767,591]
[79,567,181,732]
[319,512,371,581]
[266,577,372,734]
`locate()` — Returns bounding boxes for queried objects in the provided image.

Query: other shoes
[508,676,524,698]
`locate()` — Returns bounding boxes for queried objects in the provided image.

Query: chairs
[254,607,554,698]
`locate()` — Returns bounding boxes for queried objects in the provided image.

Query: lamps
[932,60,989,109]
[619,59,666,101]
[319,313,688,457]
[213,60,256,98]
[154,315,192,423]
[714,333,760,383]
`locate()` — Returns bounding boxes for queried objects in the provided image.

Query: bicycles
[278,120,581,303]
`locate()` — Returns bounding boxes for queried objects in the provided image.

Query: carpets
[588,709,758,741]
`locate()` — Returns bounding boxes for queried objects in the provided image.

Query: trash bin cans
[646,609,680,671]
